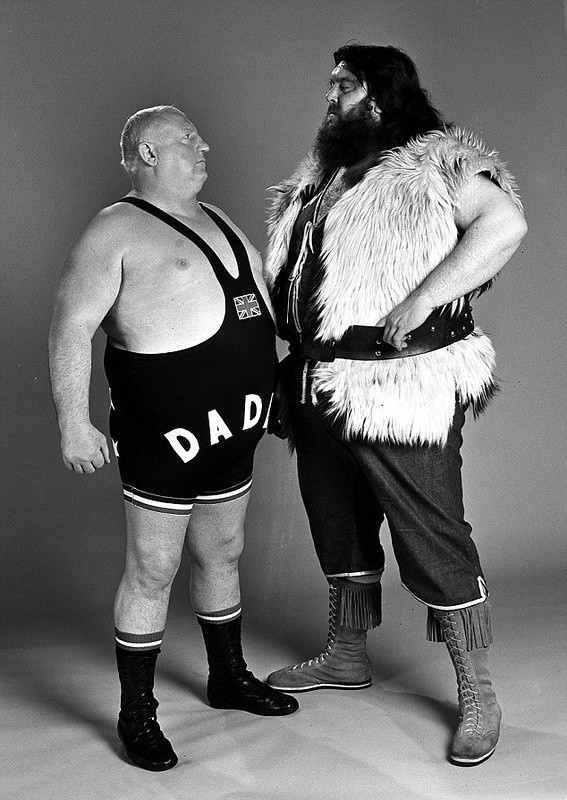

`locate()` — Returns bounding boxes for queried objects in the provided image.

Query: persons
[48,106,298,771]
[262,43,528,767]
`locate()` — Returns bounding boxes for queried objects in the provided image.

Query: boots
[197,612,300,716]
[427,600,502,767]
[115,641,178,772]
[266,577,382,690]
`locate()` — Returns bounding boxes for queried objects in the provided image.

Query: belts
[289,295,474,361]
